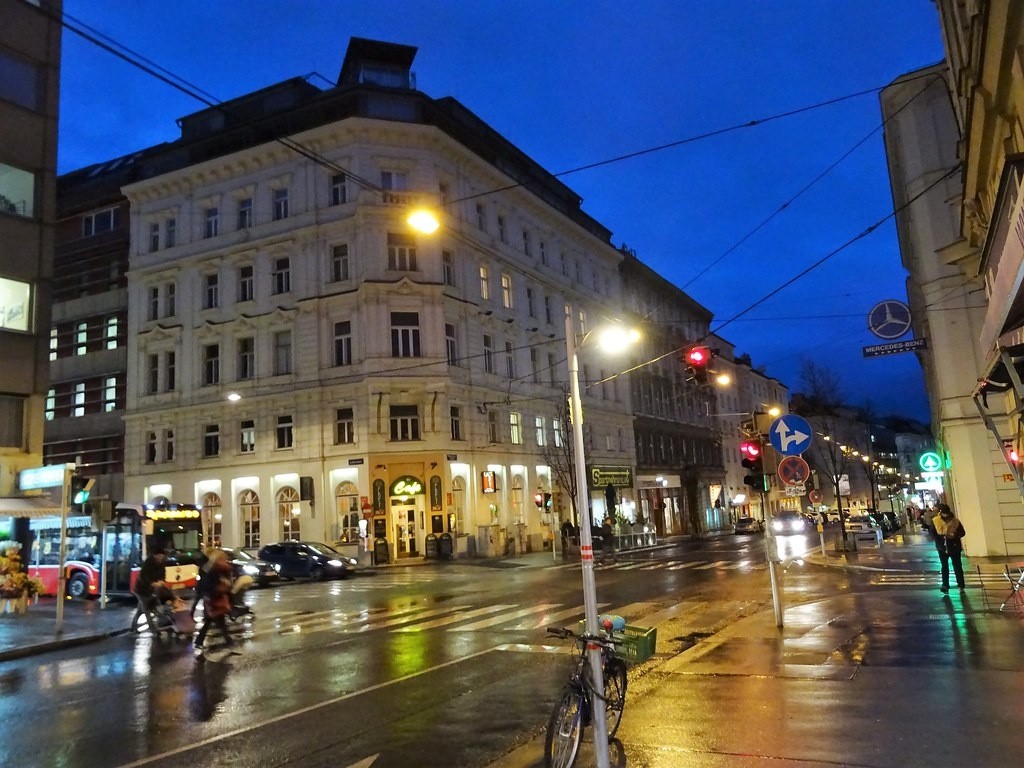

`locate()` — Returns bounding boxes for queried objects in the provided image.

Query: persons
[930,504,966,594]
[131,551,165,636]
[598,518,619,565]
[191,547,234,649]
[907,503,944,536]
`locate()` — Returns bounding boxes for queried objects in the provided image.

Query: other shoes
[195,645,203,648]
[940,586,949,594]
[959,586,964,594]
[130,628,141,633]
[150,630,161,636]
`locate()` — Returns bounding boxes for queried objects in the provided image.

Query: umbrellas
[605,482,618,535]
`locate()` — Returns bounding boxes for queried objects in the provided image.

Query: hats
[941,505,950,514]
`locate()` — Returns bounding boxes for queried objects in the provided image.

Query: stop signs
[361,504,373,519]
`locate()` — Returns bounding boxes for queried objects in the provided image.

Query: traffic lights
[739,433,768,493]
[535,493,542,507]
[70,476,91,508]
[544,493,552,513]
[82,503,95,516]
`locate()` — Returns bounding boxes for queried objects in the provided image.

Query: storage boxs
[576,614,657,662]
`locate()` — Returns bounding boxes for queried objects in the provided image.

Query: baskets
[579,615,658,663]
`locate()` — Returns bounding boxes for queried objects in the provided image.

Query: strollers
[149,584,197,636]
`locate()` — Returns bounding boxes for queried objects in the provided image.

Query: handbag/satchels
[204,595,231,618]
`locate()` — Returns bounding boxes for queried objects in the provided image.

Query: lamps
[478,310,493,315]
[547,333,556,338]
[503,319,515,323]
[375,464,387,471]
[526,328,540,332]
[431,463,437,471]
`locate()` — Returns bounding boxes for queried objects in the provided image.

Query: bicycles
[543,614,628,768]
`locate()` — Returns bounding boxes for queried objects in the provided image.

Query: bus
[0,501,205,601]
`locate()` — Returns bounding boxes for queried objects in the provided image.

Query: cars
[734,516,761,536]
[771,505,902,541]
[257,540,359,582]
[205,547,282,589]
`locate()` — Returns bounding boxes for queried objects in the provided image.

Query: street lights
[563,302,643,768]
